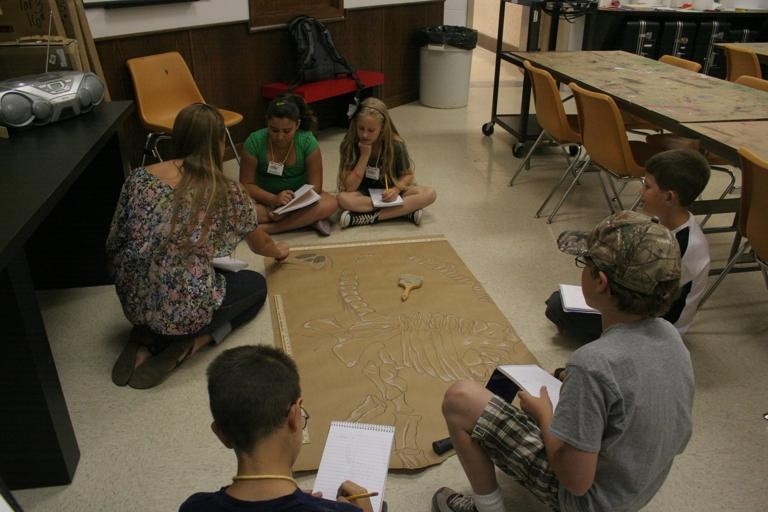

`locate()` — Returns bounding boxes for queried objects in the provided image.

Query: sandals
[113,328,142,386]
[128,338,194,389]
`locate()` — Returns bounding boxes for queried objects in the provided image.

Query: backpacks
[283,14,365,97]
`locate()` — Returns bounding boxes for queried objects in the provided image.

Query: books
[272,184,320,216]
[496,364,565,418]
[368,187,403,208]
[307,419,395,511]
[556,284,602,315]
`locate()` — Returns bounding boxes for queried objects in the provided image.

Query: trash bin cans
[419,25,477,109]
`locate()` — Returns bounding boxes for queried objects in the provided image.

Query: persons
[430,211,691,510]
[103,102,290,390]
[239,95,338,235]
[547,151,711,349]
[178,348,394,510]
[336,99,437,227]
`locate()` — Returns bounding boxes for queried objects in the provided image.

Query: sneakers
[408,210,423,225]
[432,485,478,512]
[339,209,377,228]
[315,217,330,236]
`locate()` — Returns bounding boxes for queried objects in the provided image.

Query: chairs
[724,46,762,82]
[547,81,668,227]
[506,58,584,219]
[645,74,768,229]
[127,51,245,170]
[694,150,768,313]
[618,55,702,139]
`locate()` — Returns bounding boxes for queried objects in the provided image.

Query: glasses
[301,406,310,427]
[574,252,596,277]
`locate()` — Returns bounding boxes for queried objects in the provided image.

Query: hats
[558,208,682,297]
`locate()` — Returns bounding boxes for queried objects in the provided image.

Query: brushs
[399,272,423,301]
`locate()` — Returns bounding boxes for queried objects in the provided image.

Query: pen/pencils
[343,493,378,501]
[384,173,389,194]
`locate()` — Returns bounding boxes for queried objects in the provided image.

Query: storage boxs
[697,18,731,81]
[726,28,759,42]
[622,20,659,60]
[662,22,696,61]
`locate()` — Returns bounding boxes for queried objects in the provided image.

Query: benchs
[261,72,387,129]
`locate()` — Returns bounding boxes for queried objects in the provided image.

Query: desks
[0,98,142,490]
[711,42,768,64]
[682,121,768,278]
[495,48,768,263]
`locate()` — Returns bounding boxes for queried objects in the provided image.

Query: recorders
[0,10,106,129]
